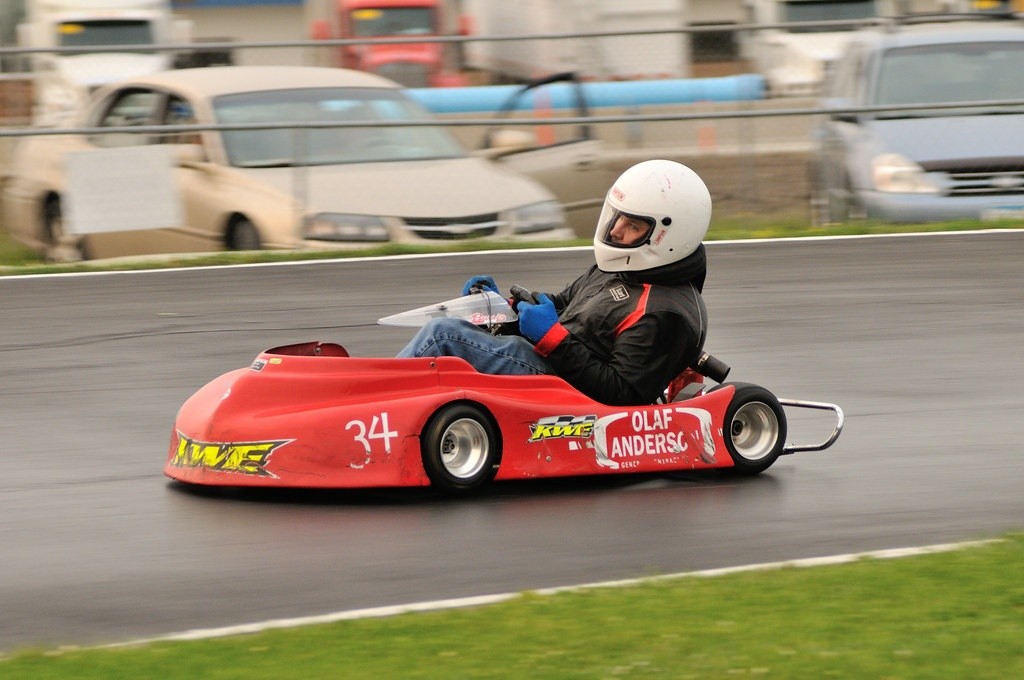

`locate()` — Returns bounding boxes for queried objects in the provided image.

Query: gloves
[515,291,559,342]
[460,276,499,294]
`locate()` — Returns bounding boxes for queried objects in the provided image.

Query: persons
[394,160,711,407]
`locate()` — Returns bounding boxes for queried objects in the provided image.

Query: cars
[3,67,597,265]
[808,14,1024,229]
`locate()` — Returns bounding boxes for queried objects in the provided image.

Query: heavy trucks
[12,0,192,123]
[308,0,485,88]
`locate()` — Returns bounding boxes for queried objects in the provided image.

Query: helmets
[593,159,711,273]
